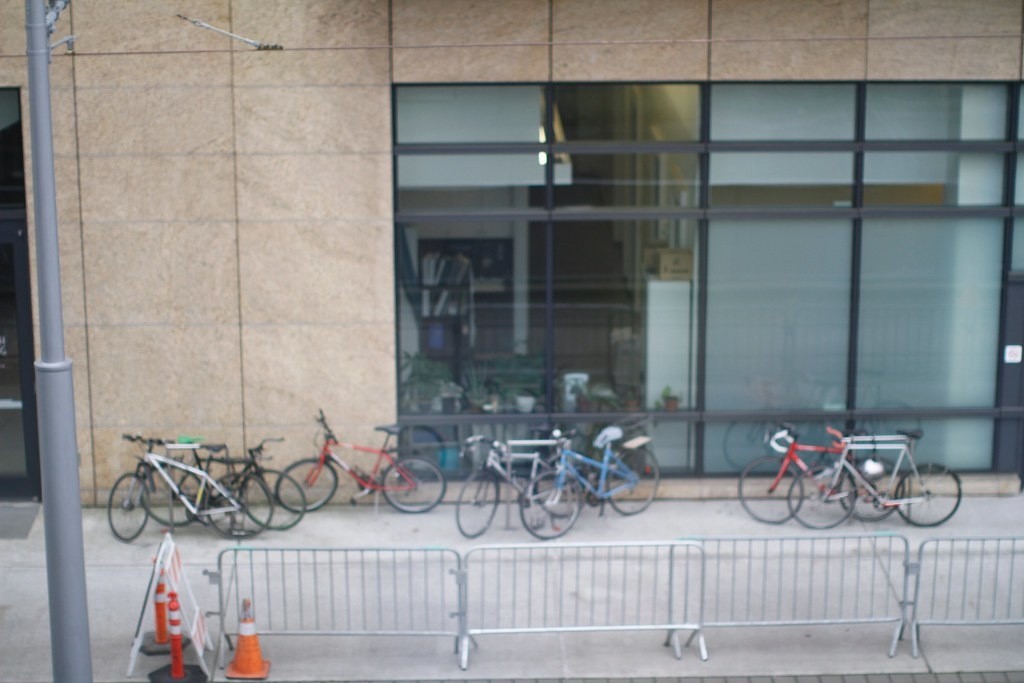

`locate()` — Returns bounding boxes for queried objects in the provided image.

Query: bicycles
[102,413,964,544]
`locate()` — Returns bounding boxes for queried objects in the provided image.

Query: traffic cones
[225,598,271,679]
